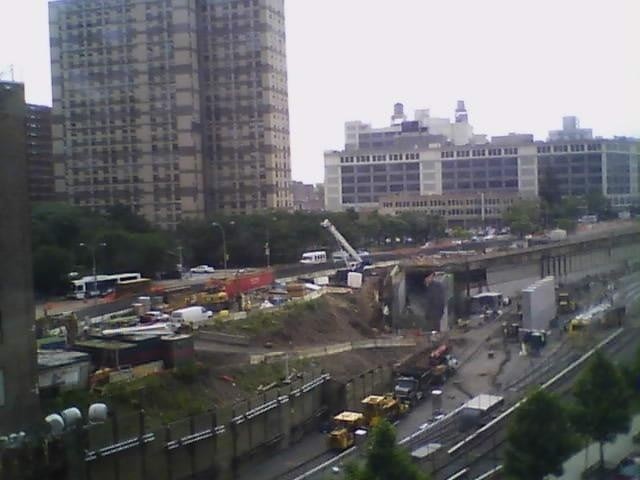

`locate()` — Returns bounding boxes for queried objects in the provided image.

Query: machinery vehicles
[320,341,507,468]
[320,219,375,279]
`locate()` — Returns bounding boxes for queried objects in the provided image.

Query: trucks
[299,251,329,266]
[62,263,328,333]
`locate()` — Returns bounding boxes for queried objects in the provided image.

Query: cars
[421,225,511,251]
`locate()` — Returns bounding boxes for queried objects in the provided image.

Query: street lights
[211,219,238,272]
[261,216,277,269]
[80,240,107,278]
